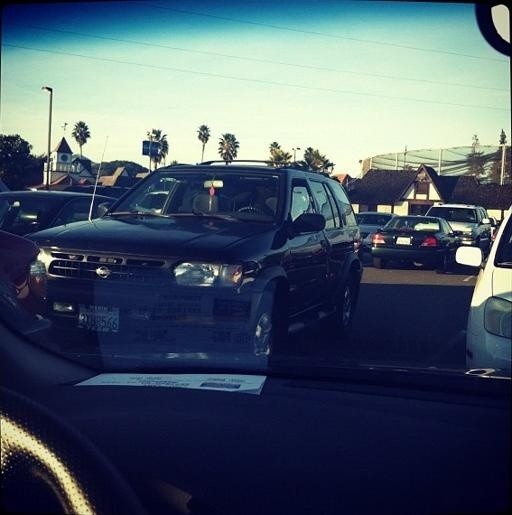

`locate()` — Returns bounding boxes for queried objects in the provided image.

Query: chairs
[265,197,279,215]
[193,193,219,215]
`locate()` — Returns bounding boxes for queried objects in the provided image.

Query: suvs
[21,161,366,373]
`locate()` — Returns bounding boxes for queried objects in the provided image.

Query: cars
[354,200,496,270]
[457,200,512,369]
[1,191,117,237]
